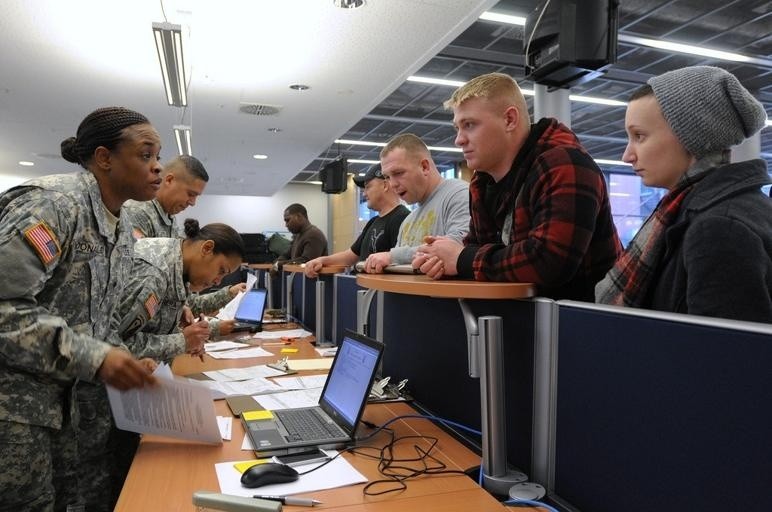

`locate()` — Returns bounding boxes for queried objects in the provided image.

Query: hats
[352,163,384,187]
[649,66,768,159]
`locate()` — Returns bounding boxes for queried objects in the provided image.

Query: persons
[182,282,246,338]
[120,156,209,362]
[365,134,472,275]
[303,162,412,278]
[270,204,329,276]
[105,220,245,493]
[0,108,161,510]
[411,73,625,303]
[594,66,771,324]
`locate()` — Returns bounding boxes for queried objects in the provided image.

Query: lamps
[268,128,281,133]
[254,154,268,159]
[152,22,188,107]
[334,1,367,7]
[291,84,308,92]
[19,161,35,165]
[173,125,191,157]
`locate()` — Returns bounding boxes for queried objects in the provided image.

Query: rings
[371,267,376,269]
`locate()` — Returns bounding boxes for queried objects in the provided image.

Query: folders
[375,378,414,402]
[183,355,298,383]
[367,375,399,404]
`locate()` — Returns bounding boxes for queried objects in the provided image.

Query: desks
[114,265,552,512]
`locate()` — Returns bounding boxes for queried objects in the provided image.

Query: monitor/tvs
[319,157,347,194]
[524,1,620,93]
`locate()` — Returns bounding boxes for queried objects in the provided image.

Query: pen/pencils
[370,263,397,270]
[253,495,324,507]
[262,342,292,345]
[198,316,204,363]
[211,315,240,327]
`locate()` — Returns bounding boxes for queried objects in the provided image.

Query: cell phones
[272,448,331,467]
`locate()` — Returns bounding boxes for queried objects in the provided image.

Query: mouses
[240,463,299,488]
[250,326,256,334]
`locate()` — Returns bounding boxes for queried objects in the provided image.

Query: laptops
[231,288,269,332]
[239,327,386,459]
